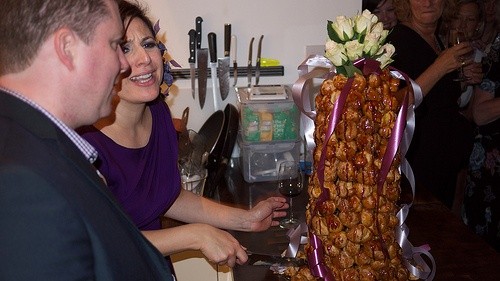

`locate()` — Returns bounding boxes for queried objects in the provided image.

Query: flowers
[324,9,395,77]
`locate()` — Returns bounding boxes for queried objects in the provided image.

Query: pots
[196,103,239,183]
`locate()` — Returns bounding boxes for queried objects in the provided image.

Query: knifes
[188,16,265,109]
[236,253,306,269]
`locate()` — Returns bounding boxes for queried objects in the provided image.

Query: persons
[76,0,289,266]
[363,1,499,235]
[1,0,176,281]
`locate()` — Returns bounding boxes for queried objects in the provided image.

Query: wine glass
[448,30,473,82]
[278,161,304,229]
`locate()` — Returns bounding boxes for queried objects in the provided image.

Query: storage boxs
[236,85,300,184]
